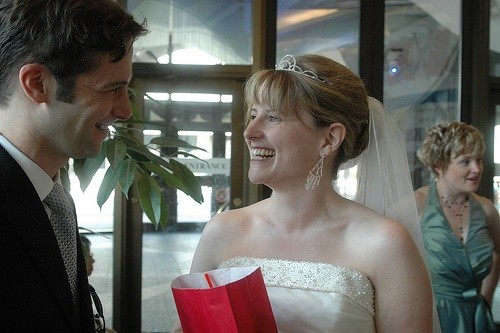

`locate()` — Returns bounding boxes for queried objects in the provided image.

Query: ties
[44,183,78,306]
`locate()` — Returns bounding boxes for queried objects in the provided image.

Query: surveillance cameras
[388,60,401,77]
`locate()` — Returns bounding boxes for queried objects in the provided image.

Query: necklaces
[437,188,470,243]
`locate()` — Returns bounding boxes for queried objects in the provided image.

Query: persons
[1,0,152,333]
[380,122,500,333]
[171,54,434,333]
